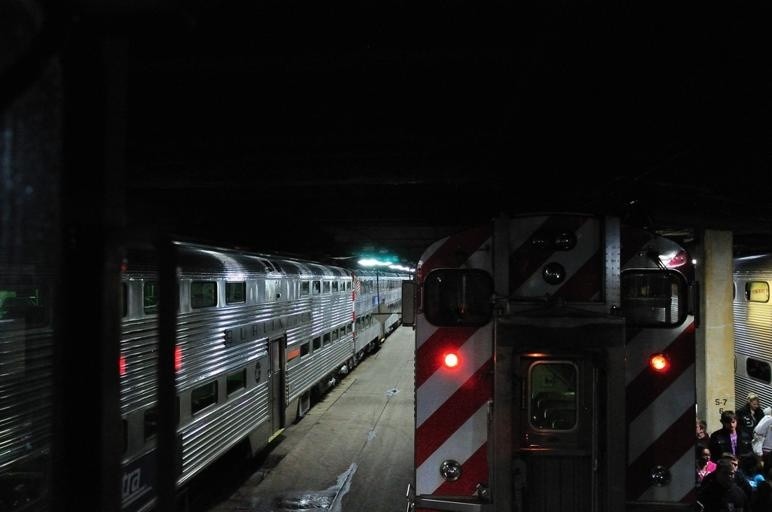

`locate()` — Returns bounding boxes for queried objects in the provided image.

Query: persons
[695,391,772,511]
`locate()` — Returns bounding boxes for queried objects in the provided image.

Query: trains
[405,205,700,511]
[732,251,771,453]
[1,236,416,510]
